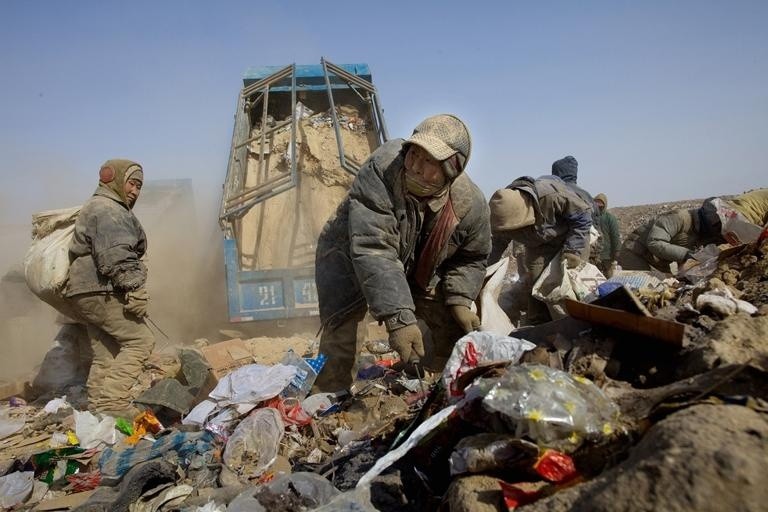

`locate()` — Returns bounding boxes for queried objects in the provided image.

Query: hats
[595,200,604,207]
[400,112,471,161]
[99,158,144,209]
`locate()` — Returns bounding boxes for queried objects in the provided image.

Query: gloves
[123,289,150,322]
[561,251,581,270]
[450,305,481,334]
[388,322,426,365]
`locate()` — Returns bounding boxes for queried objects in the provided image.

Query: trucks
[215,58,389,327]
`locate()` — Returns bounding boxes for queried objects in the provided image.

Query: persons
[309,114,492,397]
[552,155,603,264]
[489,174,599,327]
[593,193,621,280]
[63,159,151,421]
[618,198,723,272]
